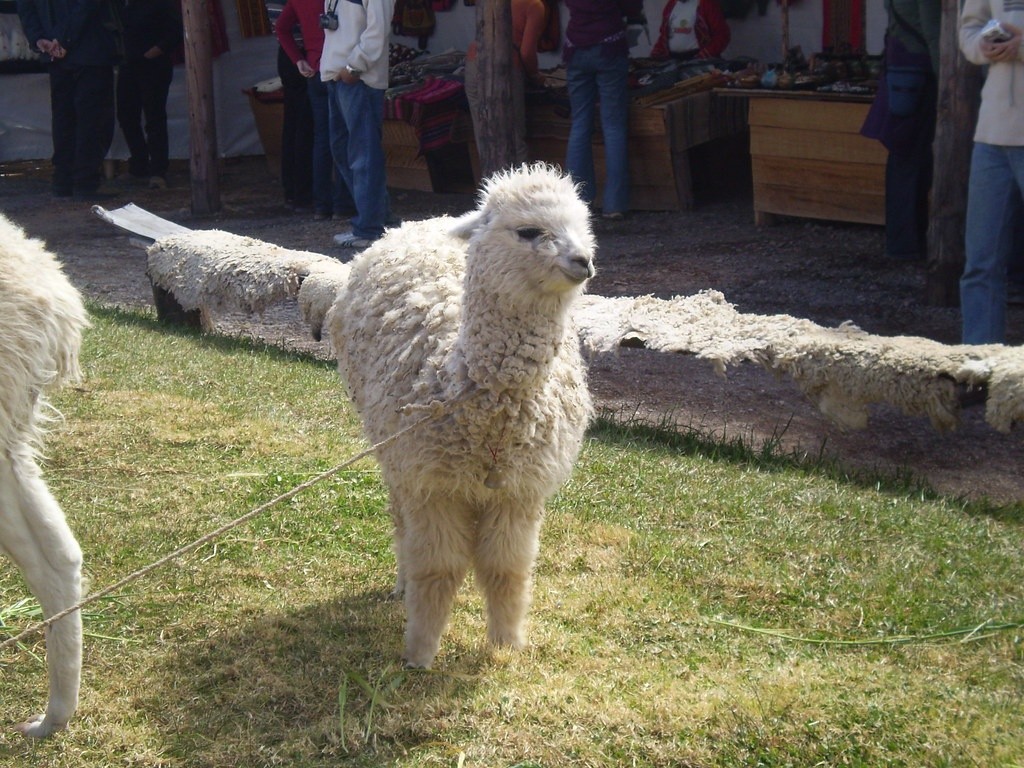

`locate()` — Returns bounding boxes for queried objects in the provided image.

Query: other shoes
[312,207,331,219]
[50,182,76,198]
[602,209,624,218]
[872,251,923,272]
[332,213,353,220]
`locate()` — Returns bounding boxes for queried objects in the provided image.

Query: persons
[957,0,1024,346]
[463,0,546,165]
[861,0,944,263]
[115,0,182,194]
[562,0,636,223]
[16,0,122,202]
[276,0,357,220]
[319,0,396,247]
[651,0,731,60]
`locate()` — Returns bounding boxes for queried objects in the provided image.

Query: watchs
[346,65,359,77]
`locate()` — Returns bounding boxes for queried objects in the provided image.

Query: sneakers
[333,230,379,247]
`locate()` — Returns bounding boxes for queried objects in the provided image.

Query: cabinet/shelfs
[712,76,887,227]
[248,88,463,194]
[0,59,114,179]
[463,83,747,211]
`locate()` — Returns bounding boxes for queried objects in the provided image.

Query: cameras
[979,19,1013,43]
[319,11,338,30]
